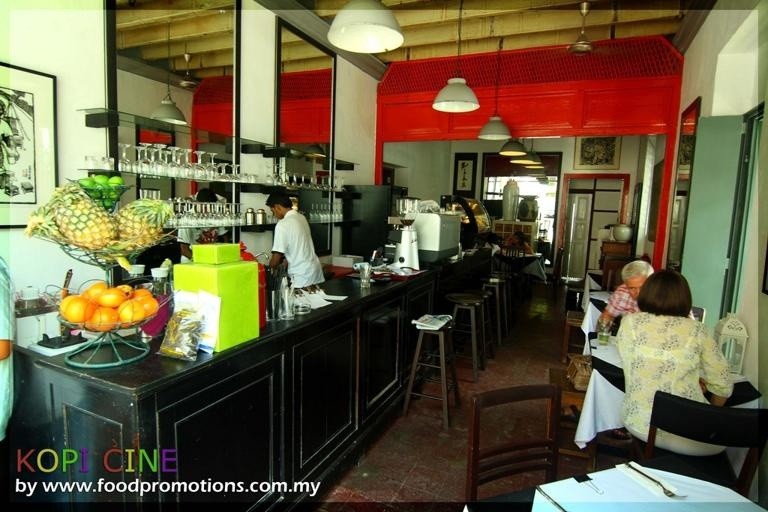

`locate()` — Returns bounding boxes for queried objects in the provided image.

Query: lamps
[149,23,189,126]
[509,156,546,176]
[179,53,197,89]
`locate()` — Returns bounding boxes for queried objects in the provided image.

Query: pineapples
[49,186,118,250]
[114,198,171,251]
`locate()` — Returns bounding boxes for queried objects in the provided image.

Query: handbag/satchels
[566,353,592,391]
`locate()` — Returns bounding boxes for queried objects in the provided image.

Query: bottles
[598,308,613,346]
[245,208,255,225]
[256,209,266,225]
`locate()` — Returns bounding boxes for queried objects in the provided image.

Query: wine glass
[192,151,207,179]
[320,176,325,188]
[273,164,278,186]
[206,153,218,180]
[131,146,144,173]
[265,165,273,185]
[118,143,131,171]
[167,147,180,177]
[300,174,306,187]
[292,173,299,186]
[309,202,342,221]
[176,151,184,165]
[180,149,192,178]
[283,172,290,186]
[166,201,244,225]
[230,164,240,181]
[219,163,230,181]
[314,176,319,188]
[154,144,168,176]
[147,147,158,175]
[309,175,314,188]
[139,143,151,174]
[162,150,171,163]
[277,167,283,186]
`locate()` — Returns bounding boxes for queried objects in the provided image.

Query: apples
[79,174,124,207]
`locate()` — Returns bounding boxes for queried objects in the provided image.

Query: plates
[293,304,311,315]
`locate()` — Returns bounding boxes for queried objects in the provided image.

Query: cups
[265,290,280,321]
[84,155,97,168]
[359,265,372,288]
[102,157,114,168]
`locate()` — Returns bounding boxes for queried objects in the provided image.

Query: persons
[265,191,326,289]
[508,231,533,254]
[597,260,695,440]
[178,187,229,263]
[616,269,734,485]
[0,255,14,441]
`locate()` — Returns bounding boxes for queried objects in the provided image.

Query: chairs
[600,258,627,292]
[465,382,562,503]
[499,246,525,273]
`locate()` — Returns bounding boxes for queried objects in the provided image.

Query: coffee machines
[383,215,460,262]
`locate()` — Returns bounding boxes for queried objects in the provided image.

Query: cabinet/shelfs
[491,219,538,253]
[598,240,631,270]
[76,107,364,228]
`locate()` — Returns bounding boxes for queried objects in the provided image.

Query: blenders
[375,197,420,271]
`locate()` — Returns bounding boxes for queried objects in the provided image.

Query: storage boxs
[173,262,261,352]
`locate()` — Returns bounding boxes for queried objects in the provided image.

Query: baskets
[40,235,175,266]
[38,277,181,331]
[66,178,136,211]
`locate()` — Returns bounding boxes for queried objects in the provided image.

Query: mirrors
[665,95,702,274]
[480,151,562,269]
[102,0,242,286]
[273,14,337,258]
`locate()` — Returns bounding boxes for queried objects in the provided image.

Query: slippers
[612,429,628,439]
[561,405,579,422]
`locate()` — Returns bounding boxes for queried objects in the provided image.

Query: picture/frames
[1,62,59,230]
[573,136,622,171]
[453,152,478,199]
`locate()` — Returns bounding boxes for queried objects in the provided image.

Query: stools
[401,271,512,431]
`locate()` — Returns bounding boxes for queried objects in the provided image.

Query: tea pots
[278,277,296,320]
